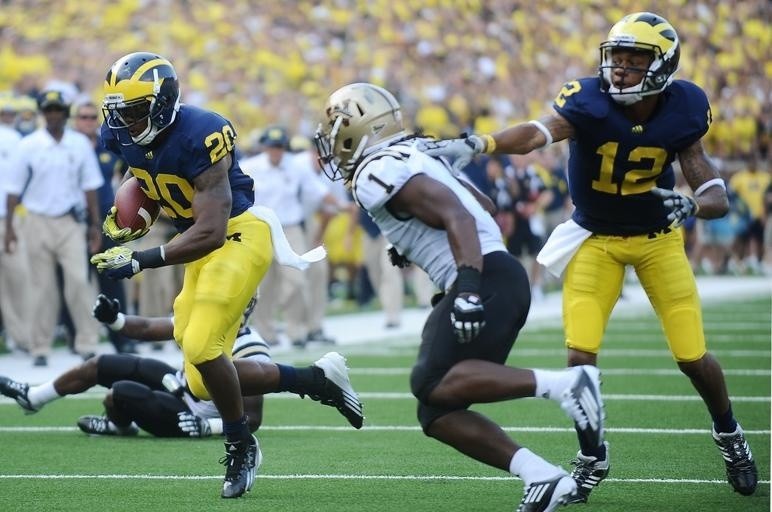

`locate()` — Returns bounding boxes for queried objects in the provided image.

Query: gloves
[90,244,165,281]
[650,187,693,230]
[449,263,487,345]
[385,243,411,270]
[419,134,483,177]
[91,293,120,324]
[101,206,151,244]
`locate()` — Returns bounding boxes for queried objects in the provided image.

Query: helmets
[314,82,406,186]
[598,11,682,106]
[100,50,182,149]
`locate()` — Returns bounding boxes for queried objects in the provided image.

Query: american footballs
[114,177,158,233]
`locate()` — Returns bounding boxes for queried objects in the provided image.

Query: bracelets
[484,133,497,156]
[108,314,128,335]
[685,194,702,221]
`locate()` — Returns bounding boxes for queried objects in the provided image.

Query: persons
[424,11,758,505]
[315,81,607,510]
[1,285,270,438]
[87,51,366,501]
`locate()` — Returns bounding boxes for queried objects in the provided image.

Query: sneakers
[562,364,608,448]
[176,411,212,437]
[514,464,579,512]
[77,415,139,436]
[570,440,611,504]
[308,351,368,430]
[219,433,263,498]
[1,375,41,413]
[712,420,758,496]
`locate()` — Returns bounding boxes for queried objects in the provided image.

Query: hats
[259,126,289,147]
[36,90,71,109]
